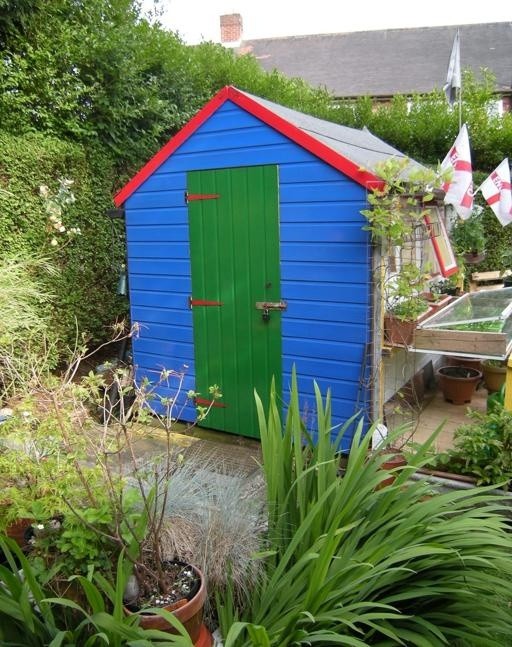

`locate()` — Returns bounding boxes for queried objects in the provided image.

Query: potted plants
[356,150,512,494]
[1,312,221,644]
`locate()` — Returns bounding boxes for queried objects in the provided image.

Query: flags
[442,28,462,111]
[478,156,511,228]
[436,120,474,224]
